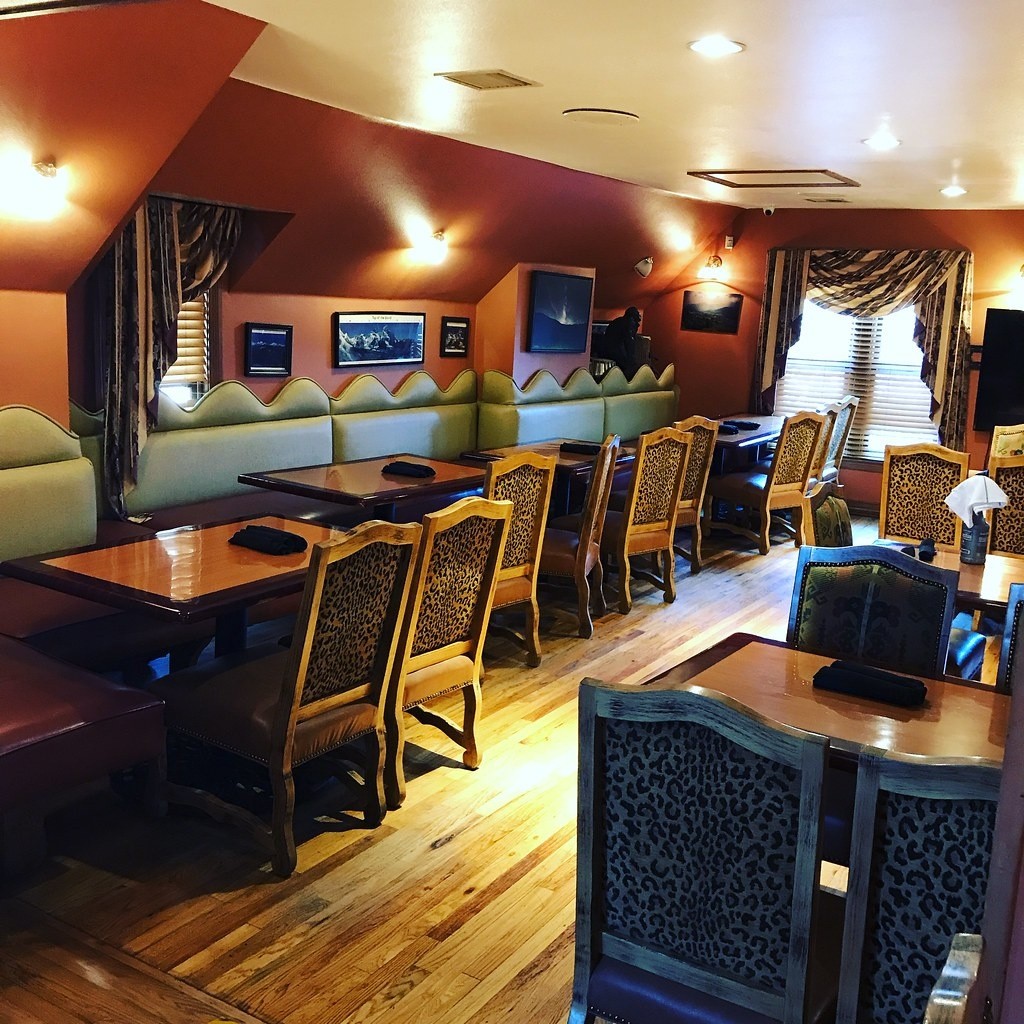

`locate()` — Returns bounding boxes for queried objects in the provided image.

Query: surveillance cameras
[763,207,775,216]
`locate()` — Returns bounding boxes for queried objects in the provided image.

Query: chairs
[163,393,1023,1023]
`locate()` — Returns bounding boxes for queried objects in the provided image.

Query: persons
[603,306,641,382]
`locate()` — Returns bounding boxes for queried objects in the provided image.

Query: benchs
[0,359,685,810]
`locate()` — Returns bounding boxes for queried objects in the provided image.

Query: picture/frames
[332,311,426,367]
[441,315,471,358]
[527,269,592,354]
[242,320,293,377]
[681,288,744,336]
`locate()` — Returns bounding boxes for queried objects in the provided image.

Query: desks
[632,630,1013,878]
[1,513,354,817]
[860,538,1024,630]
[238,451,486,521]
[711,413,785,536]
[459,435,637,516]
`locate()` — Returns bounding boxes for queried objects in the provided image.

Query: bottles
[959,510,990,565]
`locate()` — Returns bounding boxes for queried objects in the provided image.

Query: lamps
[938,165,969,197]
[699,256,723,276]
[635,258,653,279]
[34,160,58,179]
[432,230,446,242]
[862,121,898,149]
